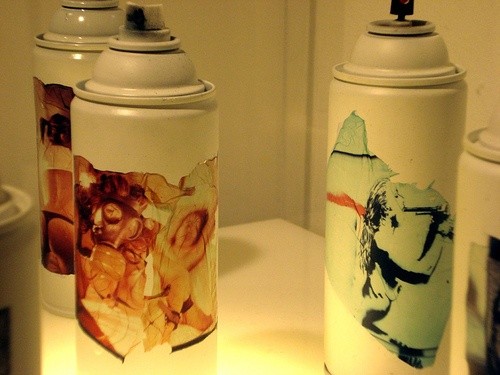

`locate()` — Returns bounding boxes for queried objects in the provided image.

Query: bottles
[458,119,499,375]
[70,2,216,375]
[0,187,43,375]
[325,0,467,375]
[34,1,122,317]
